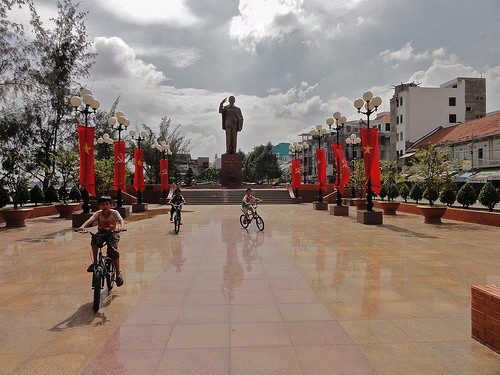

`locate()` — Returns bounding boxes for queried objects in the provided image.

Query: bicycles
[74,228,127,315]
[239,201,265,231]
[168,203,186,234]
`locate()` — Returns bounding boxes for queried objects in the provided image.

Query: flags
[133,148,146,192]
[291,159,302,192]
[113,141,126,190]
[314,147,327,192]
[359,127,385,197]
[330,143,350,193]
[159,158,169,193]
[77,124,97,197]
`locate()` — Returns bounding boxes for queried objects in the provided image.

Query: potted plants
[408,141,466,225]
[349,159,367,210]
[90,171,106,211]
[0,160,37,228]
[55,186,81,219]
[377,159,404,215]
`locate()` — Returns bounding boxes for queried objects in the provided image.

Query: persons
[76,196,125,287]
[166,188,187,225]
[218,95,244,154]
[241,188,262,224]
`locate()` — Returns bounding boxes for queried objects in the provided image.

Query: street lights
[353,90,383,212]
[326,111,347,206]
[288,141,309,184]
[346,134,361,162]
[128,129,148,204]
[152,140,175,206]
[69,88,101,213]
[97,133,114,161]
[309,124,328,202]
[108,111,131,206]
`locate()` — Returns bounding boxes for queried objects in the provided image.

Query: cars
[263,182,291,187]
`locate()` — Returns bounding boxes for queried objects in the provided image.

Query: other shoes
[180,222,182,225]
[170,216,172,221]
[243,220,246,224]
[116,274,123,286]
[87,263,94,272]
